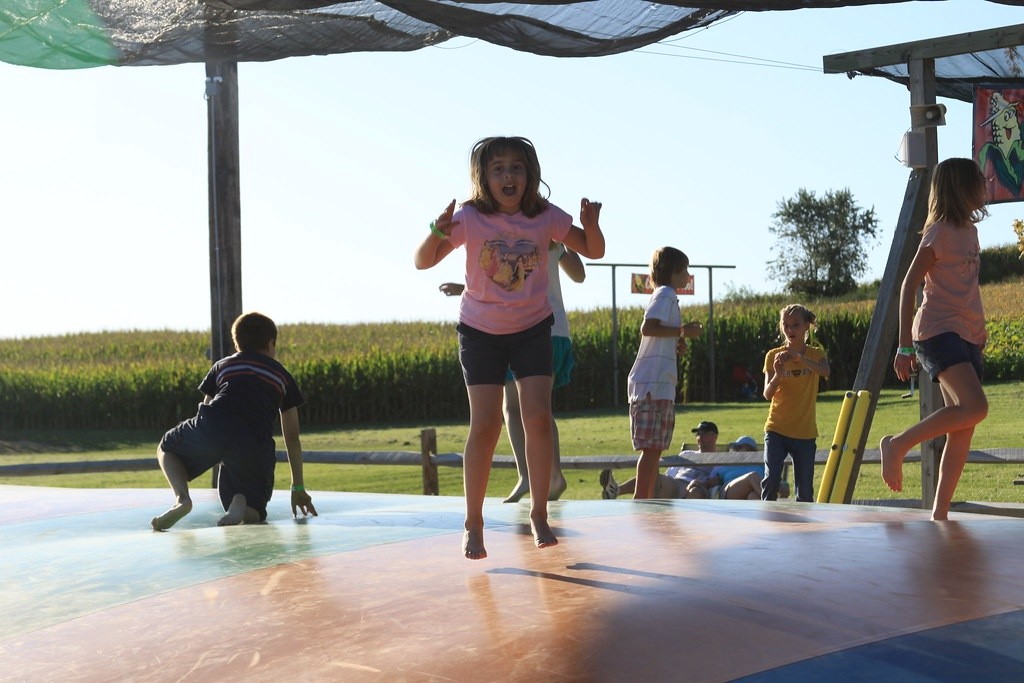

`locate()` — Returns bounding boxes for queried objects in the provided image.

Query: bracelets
[681,326,684,337]
[290,485,305,491]
[429,220,451,240]
[794,353,801,362]
[897,347,915,356]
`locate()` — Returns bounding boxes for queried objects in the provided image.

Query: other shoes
[777,480,790,502]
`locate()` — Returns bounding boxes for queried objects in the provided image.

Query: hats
[692,422,718,434]
[728,436,758,451]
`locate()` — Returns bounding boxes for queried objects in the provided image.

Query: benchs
[682,443,789,483]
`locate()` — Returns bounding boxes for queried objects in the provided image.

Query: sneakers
[600,468,618,499]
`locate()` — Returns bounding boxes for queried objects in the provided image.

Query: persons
[683,436,789,500]
[414,136,605,560]
[438,237,585,503]
[627,246,703,500]
[881,158,989,520]
[150,313,318,530]
[600,421,719,498]
[761,304,830,503]
